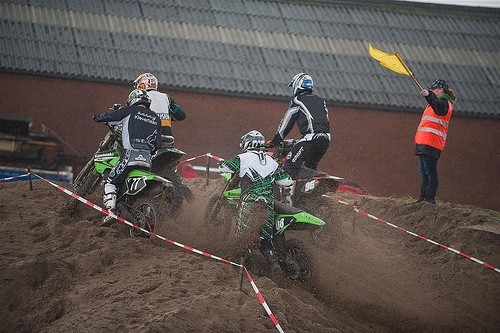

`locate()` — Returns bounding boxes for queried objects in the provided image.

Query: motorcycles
[69,104,188,240]
[263,138,344,252]
[204,162,326,292]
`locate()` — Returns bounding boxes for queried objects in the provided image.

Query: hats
[427,79,449,89]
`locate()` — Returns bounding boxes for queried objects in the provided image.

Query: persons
[131,72,186,147]
[411,78,456,204]
[92,88,164,228]
[218,130,295,288]
[267,73,331,177]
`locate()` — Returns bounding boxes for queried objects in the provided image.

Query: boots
[99,194,120,228]
[259,240,283,284]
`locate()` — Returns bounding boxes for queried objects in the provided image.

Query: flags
[369,43,413,77]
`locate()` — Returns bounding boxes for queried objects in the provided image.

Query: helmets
[125,89,151,107]
[240,130,266,154]
[287,72,314,96]
[132,73,157,90]
